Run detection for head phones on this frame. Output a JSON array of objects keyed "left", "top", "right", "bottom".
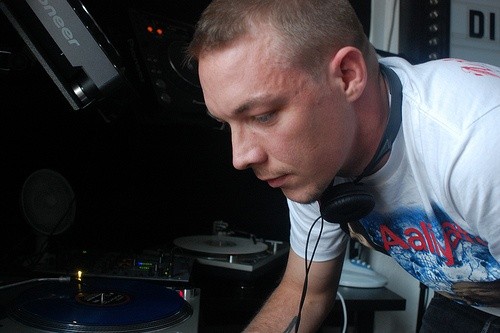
[{"left": 319, "top": 62, "right": 403, "bottom": 224}]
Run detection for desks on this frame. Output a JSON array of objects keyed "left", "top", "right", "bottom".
[{"left": 328, "top": 286, "right": 407, "bottom": 333}]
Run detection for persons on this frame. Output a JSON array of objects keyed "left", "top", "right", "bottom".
[{"left": 189, "top": 0, "right": 500, "bottom": 333}]
